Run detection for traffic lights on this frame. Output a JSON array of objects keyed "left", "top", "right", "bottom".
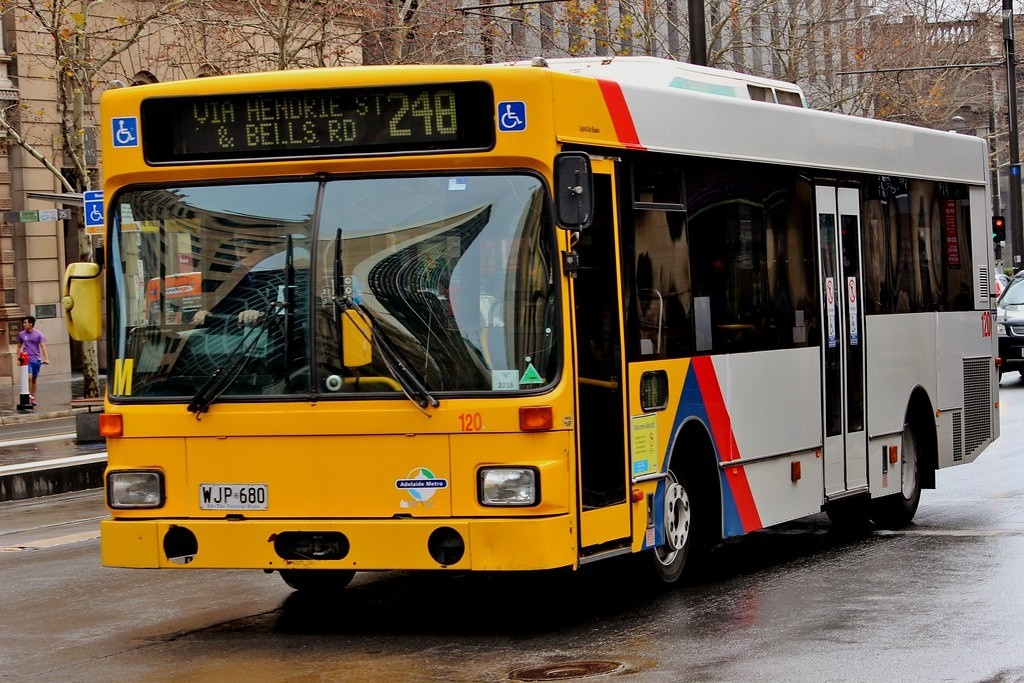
[{"left": 992, "top": 216, "right": 1006, "bottom": 242}]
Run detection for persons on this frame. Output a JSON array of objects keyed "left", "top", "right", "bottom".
[
  {"left": 16, "top": 315, "right": 49, "bottom": 405},
  {"left": 1004, "top": 268, "right": 1015, "bottom": 282},
  {"left": 192, "top": 222, "right": 288, "bottom": 329}
]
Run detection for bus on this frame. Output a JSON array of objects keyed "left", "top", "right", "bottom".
[
  {"left": 61, "top": 56, "right": 1001, "bottom": 589},
  {"left": 146, "top": 272, "right": 201, "bottom": 325}
]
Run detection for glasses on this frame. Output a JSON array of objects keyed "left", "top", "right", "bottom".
[{"left": 235, "top": 238, "right": 264, "bottom": 253}]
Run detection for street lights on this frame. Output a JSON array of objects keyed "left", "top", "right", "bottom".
[{"left": 952, "top": 116, "right": 977, "bottom": 136}]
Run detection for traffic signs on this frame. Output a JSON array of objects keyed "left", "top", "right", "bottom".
[{"left": 320, "top": 276, "right": 354, "bottom": 307}]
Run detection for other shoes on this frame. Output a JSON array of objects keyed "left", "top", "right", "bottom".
[{"left": 29, "top": 397, "right": 37, "bottom": 406}]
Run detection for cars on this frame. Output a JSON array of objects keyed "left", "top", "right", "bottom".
[{"left": 996, "top": 270, "right": 1024, "bottom": 382}]
